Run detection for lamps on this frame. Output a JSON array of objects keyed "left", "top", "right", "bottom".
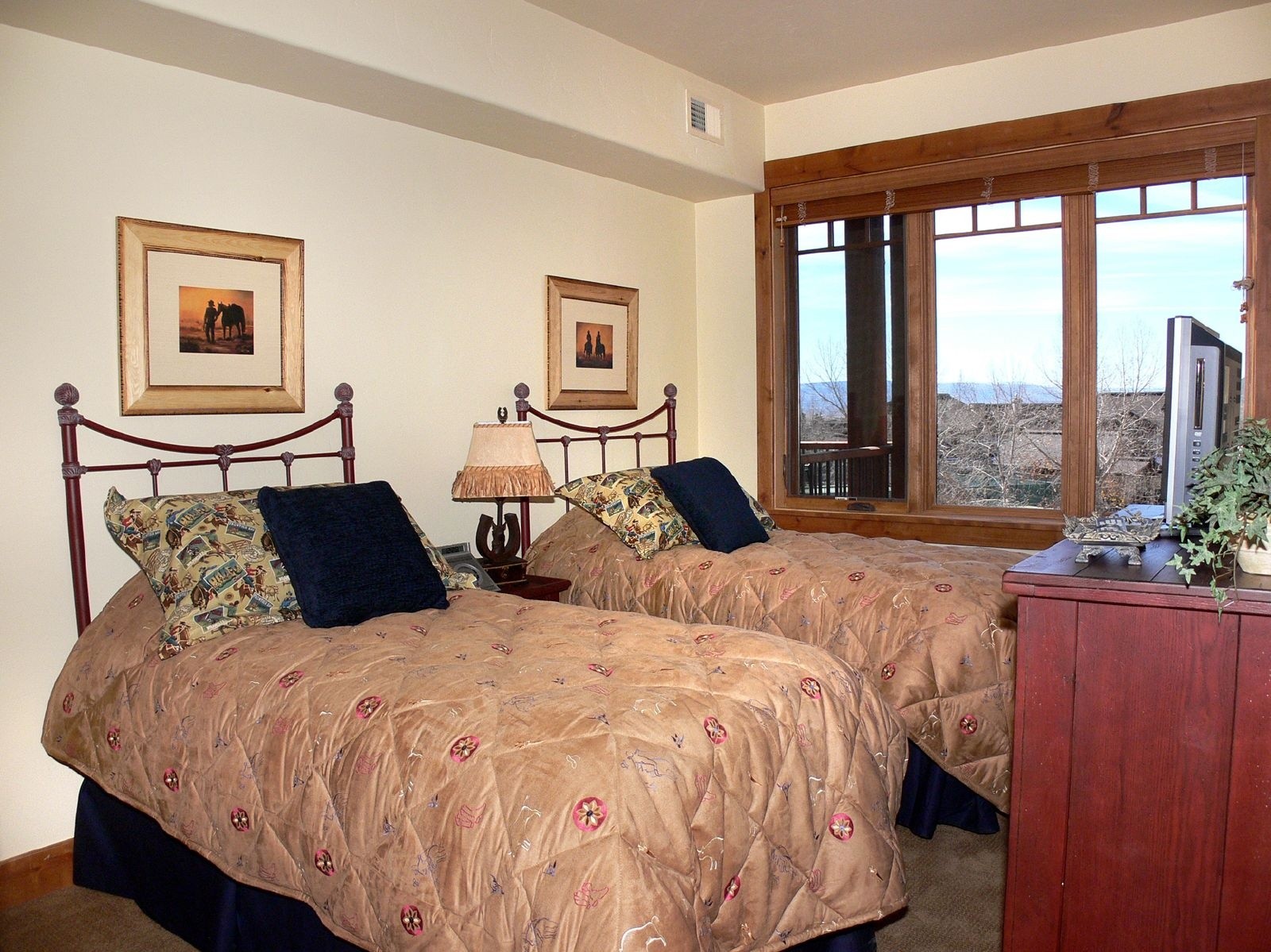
[{"left": 451, "top": 422, "right": 556, "bottom": 588}]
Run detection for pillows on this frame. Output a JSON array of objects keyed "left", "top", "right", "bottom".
[
  {"left": 556, "top": 466, "right": 779, "bottom": 556},
  {"left": 103, "top": 483, "right": 476, "bottom": 662},
  {"left": 260, "top": 482, "right": 450, "bottom": 629},
  {"left": 650, "top": 458, "right": 771, "bottom": 554}
]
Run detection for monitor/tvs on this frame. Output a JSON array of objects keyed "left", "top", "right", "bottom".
[{"left": 1147, "top": 313, "right": 1242, "bottom": 537}]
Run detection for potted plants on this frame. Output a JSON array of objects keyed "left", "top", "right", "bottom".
[{"left": 1169, "top": 419, "right": 1271, "bottom": 606}]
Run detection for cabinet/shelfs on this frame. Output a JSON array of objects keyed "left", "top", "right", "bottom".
[{"left": 1001, "top": 504, "right": 1270, "bottom": 952}]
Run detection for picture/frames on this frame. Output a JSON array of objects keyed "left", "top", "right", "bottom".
[
  {"left": 114, "top": 214, "right": 309, "bottom": 419},
  {"left": 547, "top": 276, "right": 639, "bottom": 412}
]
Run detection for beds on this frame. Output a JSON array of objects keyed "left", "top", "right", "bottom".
[
  {"left": 515, "top": 382, "right": 1044, "bottom": 841},
  {"left": 53, "top": 382, "right": 911, "bottom": 952}
]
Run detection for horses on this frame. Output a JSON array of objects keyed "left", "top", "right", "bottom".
[
  {"left": 585, "top": 335, "right": 592, "bottom": 357},
  {"left": 595, "top": 336, "right": 605, "bottom": 361},
  {"left": 216, "top": 293, "right": 246, "bottom": 339}
]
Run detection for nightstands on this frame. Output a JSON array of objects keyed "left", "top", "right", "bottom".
[{"left": 497, "top": 575, "right": 572, "bottom": 602}]
